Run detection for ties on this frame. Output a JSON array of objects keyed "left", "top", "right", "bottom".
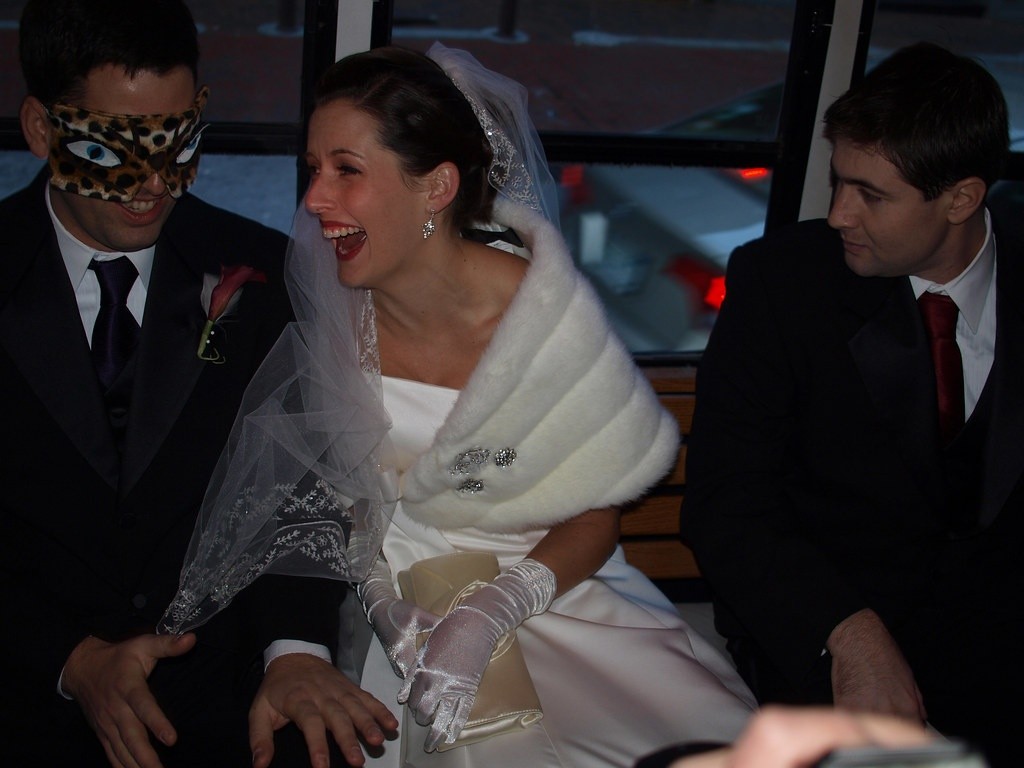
[
  {"left": 918, "top": 291, "right": 965, "bottom": 446},
  {"left": 88, "top": 256, "right": 141, "bottom": 387}
]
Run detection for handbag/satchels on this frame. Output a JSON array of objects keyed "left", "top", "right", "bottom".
[{"left": 398, "top": 551, "right": 544, "bottom": 752}]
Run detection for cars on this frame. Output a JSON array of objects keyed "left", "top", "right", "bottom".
[
  {"left": 631, "top": 50, "right": 1024, "bottom": 187},
  {"left": 536, "top": 165, "right": 771, "bottom": 361}
]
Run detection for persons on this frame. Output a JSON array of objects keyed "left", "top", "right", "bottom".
[
  {"left": 0, "top": 0, "right": 399, "bottom": 768},
  {"left": 681, "top": 43, "right": 1023, "bottom": 768},
  {"left": 168, "top": 42, "right": 759, "bottom": 768}
]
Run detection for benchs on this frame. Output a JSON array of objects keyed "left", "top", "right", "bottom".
[{"left": 613, "top": 365, "right": 704, "bottom": 578}]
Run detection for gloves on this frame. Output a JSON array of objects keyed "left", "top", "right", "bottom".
[
  {"left": 346, "top": 530, "right": 443, "bottom": 678},
  {"left": 397, "top": 558, "right": 558, "bottom": 754}
]
